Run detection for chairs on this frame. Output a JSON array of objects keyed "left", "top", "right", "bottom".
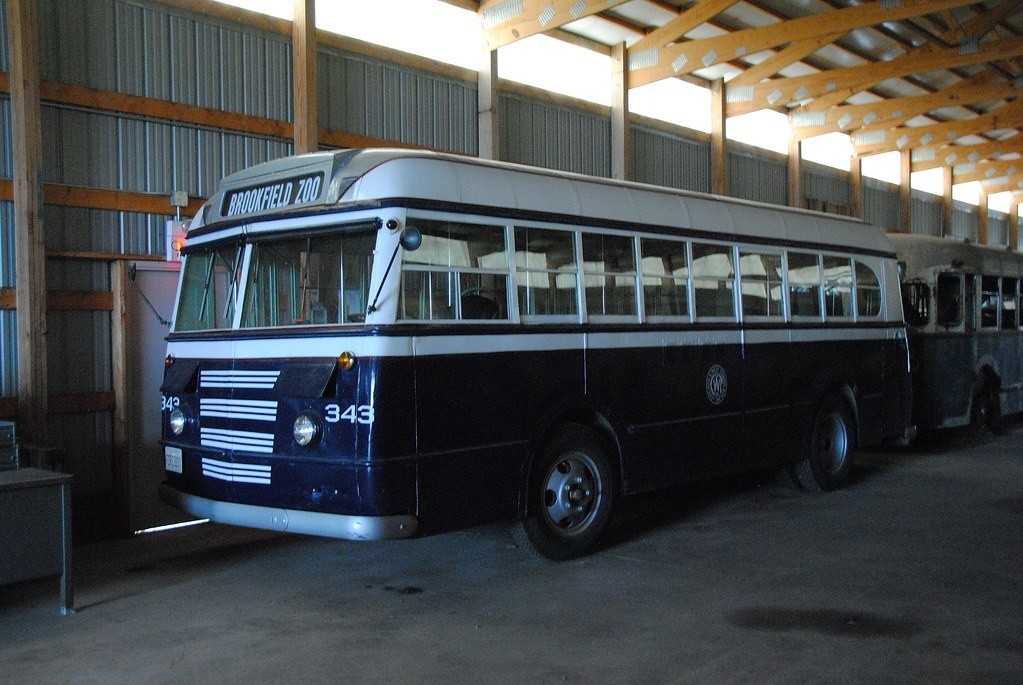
[{"left": 442, "top": 286, "right": 505, "bottom": 320}]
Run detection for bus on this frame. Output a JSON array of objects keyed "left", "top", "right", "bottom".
[
  {"left": 127, "top": 145, "right": 916, "bottom": 562},
  {"left": 884, "top": 232, "right": 1023, "bottom": 448}
]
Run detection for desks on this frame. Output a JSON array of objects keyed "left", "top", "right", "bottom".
[{"left": 0, "top": 468, "right": 76, "bottom": 616}]
